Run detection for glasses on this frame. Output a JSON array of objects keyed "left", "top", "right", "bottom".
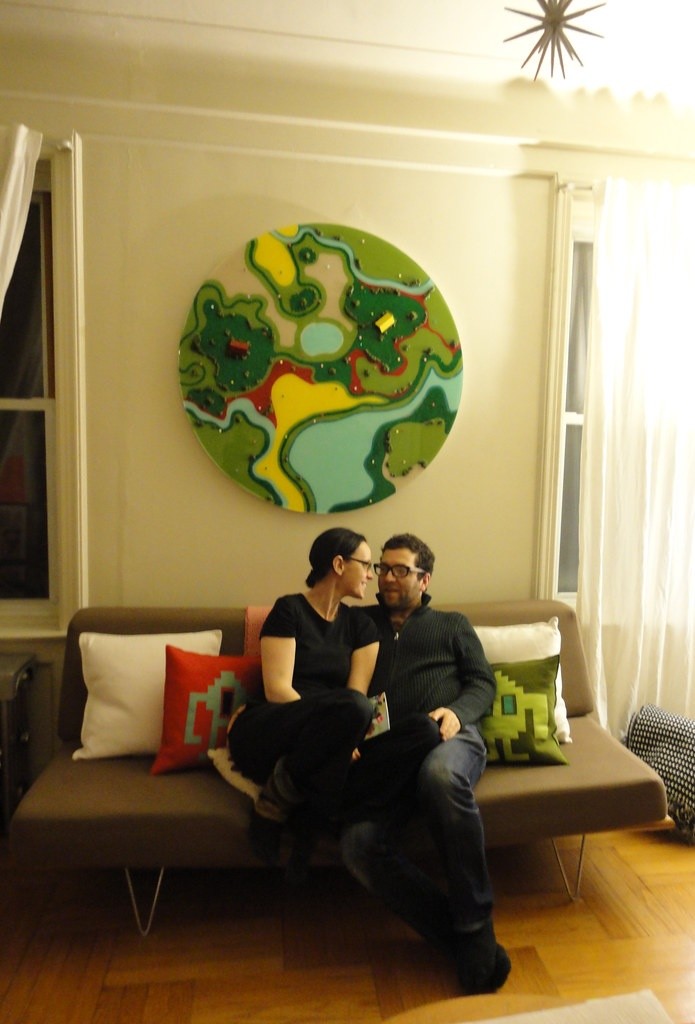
[
  {"left": 374, "top": 563, "right": 426, "bottom": 578},
  {"left": 347, "top": 557, "right": 371, "bottom": 571}
]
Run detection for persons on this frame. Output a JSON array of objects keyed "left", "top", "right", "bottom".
[
  {"left": 226, "top": 527, "right": 381, "bottom": 872},
  {"left": 226, "top": 533, "right": 511, "bottom": 993}
]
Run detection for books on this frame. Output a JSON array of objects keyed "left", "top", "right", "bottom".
[{"left": 363, "top": 692, "right": 390, "bottom": 741}]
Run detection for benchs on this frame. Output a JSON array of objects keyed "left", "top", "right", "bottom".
[{"left": 7, "top": 602, "right": 676, "bottom": 938}]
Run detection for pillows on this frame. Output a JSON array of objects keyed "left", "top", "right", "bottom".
[
  {"left": 149, "top": 645, "right": 262, "bottom": 775},
  {"left": 72, "top": 630, "right": 223, "bottom": 759},
  {"left": 474, "top": 617, "right": 573, "bottom": 763}
]
